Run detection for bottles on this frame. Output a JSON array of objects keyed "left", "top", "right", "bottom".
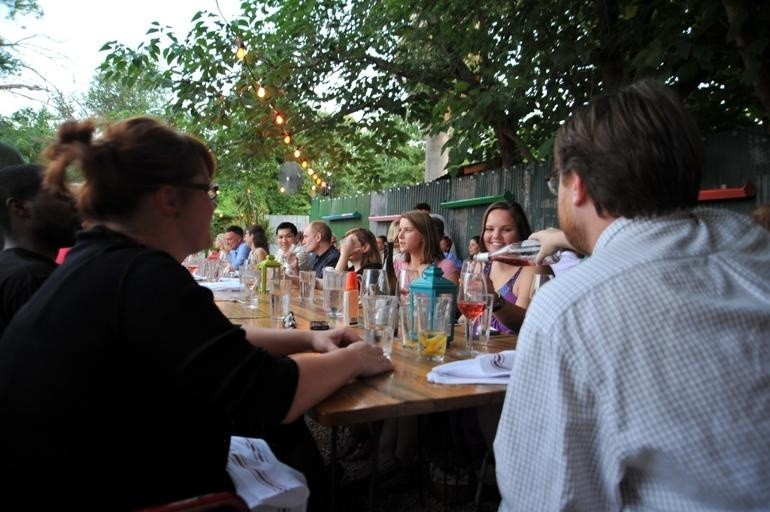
[
  {"left": 471, "top": 237, "right": 562, "bottom": 266},
  {"left": 343, "top": 272, "right": 359, "bottom": 327}
]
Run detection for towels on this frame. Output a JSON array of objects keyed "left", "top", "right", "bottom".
[
  {"left": 419, "top": 350, "right": 517, "bottom": 386},
  {"left": 225, "top": 433, "right": 312, "bottom": 512}
]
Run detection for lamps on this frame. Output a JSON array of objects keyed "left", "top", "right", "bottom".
[{"left": 409, "top": 262, "right": 458, "bottom": 348}]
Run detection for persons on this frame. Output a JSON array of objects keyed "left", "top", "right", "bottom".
[
  {"left": 0, "top": 114, "right": 396, "bottom": 511},
  {"left": 215, "top": 204, "right": 462, "bottom": 322},
  {"left": 490, "top": 79, "right": 770, "bottom": 512},
  {"left": 0, "top": 141, "right": 89, "bottom": 328},
  {"left": 459, "top": 200, "right": 549, "bottom": 339}
]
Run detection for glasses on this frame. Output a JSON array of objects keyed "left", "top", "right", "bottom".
[
  {"left": 544, "top": 170, "right": 559, "bottom": 197},
  {"left": 169, "top": 180, "right": 219, "bottom": 200}
]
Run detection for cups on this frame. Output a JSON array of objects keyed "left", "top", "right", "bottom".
[
  {"left": 469, "top": 291, "right": 494, "bottom": 352},
  {"left": 186, "top": 250, "right": 341, "bottom": 324},
  {"left": 363, "top": 292, "right": 453, "bottom": 365}
]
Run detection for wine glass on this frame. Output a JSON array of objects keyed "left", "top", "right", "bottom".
[
  {"left": 457, "top": 273, "right": 485, "bottom": 358},
  {"left": 395, "top": 268, "right": 421, "bottom": 302},
  {"left": 360, "top": 268, "right": 390, "bottom": 298}
]
